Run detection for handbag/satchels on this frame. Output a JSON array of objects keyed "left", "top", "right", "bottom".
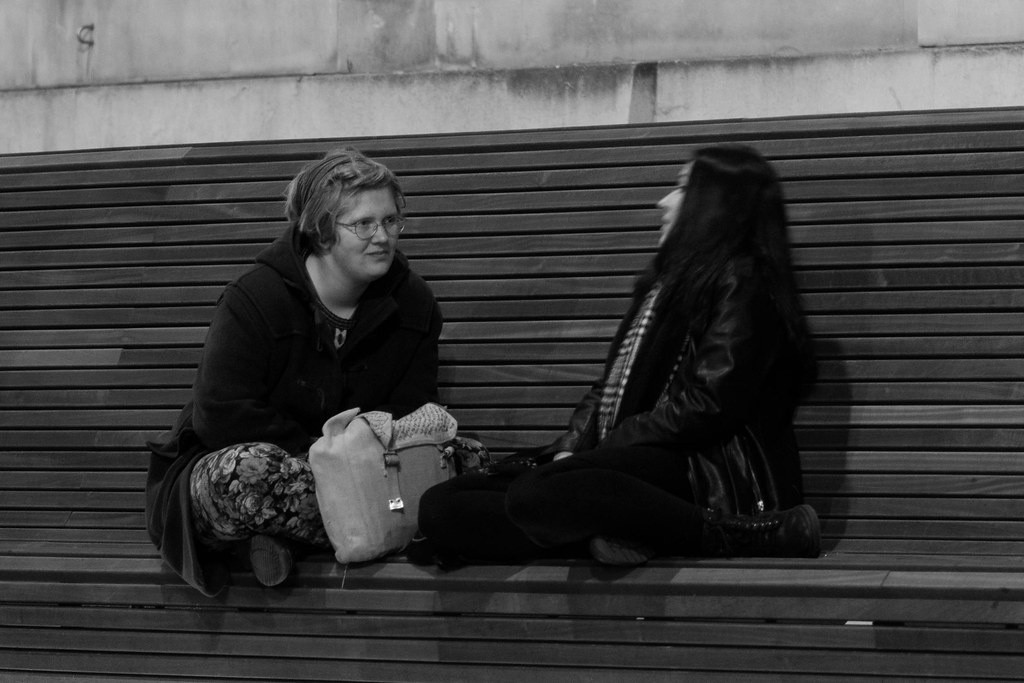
[{"left": 308, "top": 401, "right": 459, "bottom": 565}]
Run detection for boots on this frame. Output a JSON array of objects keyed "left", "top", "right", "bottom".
[
  {"left": 697, "top": 497, "right": 821, "bottom": 559},
  {"left": 587, "top": 536, "right": 647, "bottom": 566}
]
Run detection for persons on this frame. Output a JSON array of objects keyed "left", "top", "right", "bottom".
[
  {"left": 146, "top": 145, "right": 443, "bottom": 597},
  {"left": 543, "top": 146, "right": 822, "bottom": 563}
]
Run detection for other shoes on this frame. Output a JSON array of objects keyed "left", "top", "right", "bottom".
[{"left": 250, "top": 534, "right": 293, "bottom": 587}]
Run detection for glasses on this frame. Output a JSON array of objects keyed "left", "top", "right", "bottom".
[{"left": 335, "top": 214, "right": 408, "bottom": 240}]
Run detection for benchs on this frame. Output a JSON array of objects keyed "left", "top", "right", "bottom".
[{"left": 0, "top": 106, "right": 1024, "bottom": 683}]
